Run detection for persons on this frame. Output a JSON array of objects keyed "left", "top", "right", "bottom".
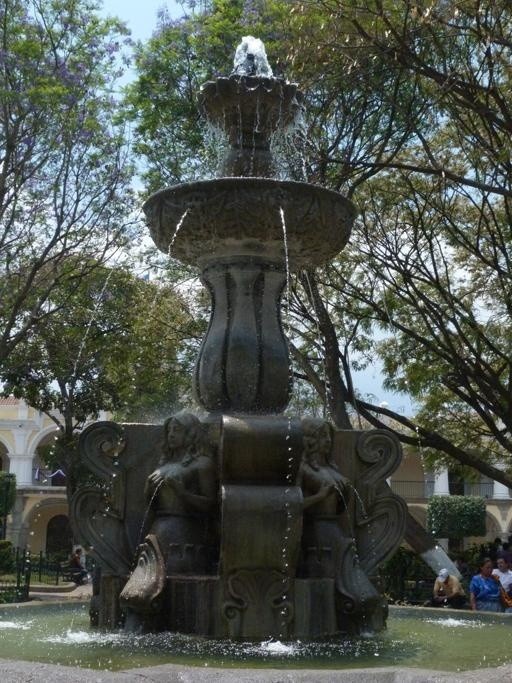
[
  {"left": 423, "top": 567, "right": 466, "bottom": 609},
  {"left": 295, "top": 417, "right": 379, "bottom": 632},
  {"left": 69, "top": 547, "right": 84, "bottom": 569},
  {"left": 118, "top": 411, "right": 217, "bottom": 634},
  {"left": 469, "top": 557, "right": 511, "bottom": 611},
  {"left": 491, "top": 556, "right": 512, "bottom": 612}
]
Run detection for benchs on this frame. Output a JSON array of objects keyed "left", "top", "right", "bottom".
[{"left": 60, "top": 555, "right": 93, "bottom": 586}]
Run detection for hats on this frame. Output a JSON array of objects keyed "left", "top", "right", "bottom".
[{"left": 438, "top": 568, "right": 450, "bottom": 583}]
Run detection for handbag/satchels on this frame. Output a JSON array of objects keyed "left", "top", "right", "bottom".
[{"left": 498, "top": 585, "right": 512, "bottom": 609}]
